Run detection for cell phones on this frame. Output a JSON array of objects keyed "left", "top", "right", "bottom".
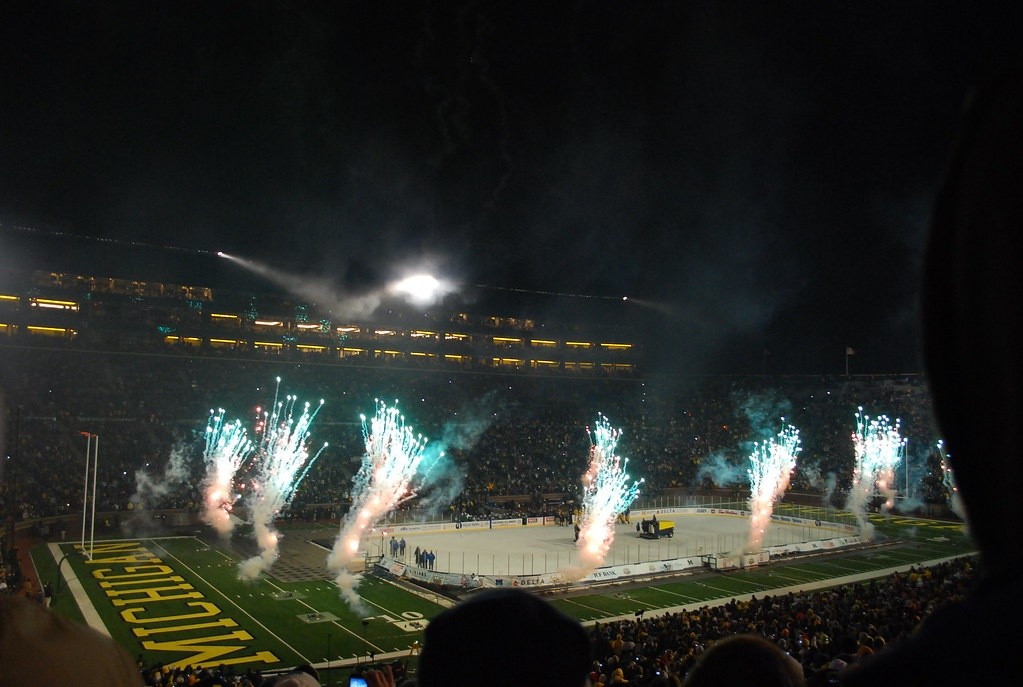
[{"left": 347, "top": 674, "right": 368, "bottom": 687}]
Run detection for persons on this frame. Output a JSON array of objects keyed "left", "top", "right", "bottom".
[{"left": 0, "top": 90, "right": 1022, "bottom": 687}]
